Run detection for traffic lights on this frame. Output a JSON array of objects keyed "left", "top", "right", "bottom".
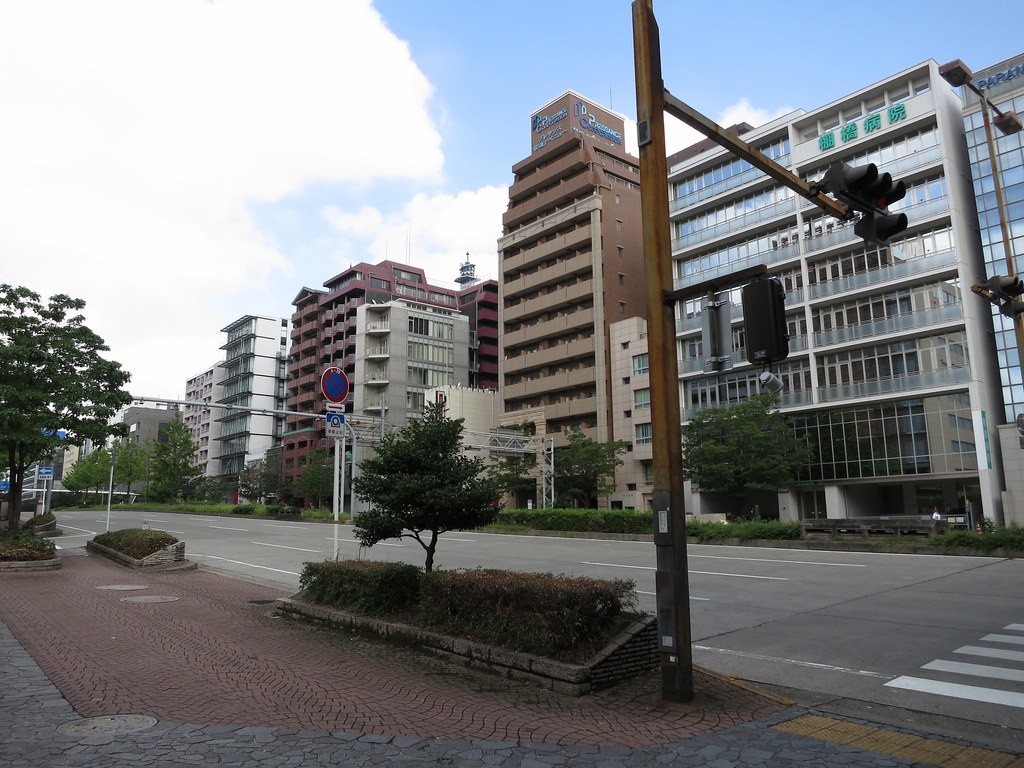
[
  {"left": 994, "top": 275, "right": 1024, "bottom": 301},
  {"left": 837, "top": 159, "right": 907, "bottom": 212},
  {"left": 869, "top": 211, "right": 908, "bottom": 247},
  {"left": 1011, "top": 301, "right": 1024, "bottom": 318}
]
